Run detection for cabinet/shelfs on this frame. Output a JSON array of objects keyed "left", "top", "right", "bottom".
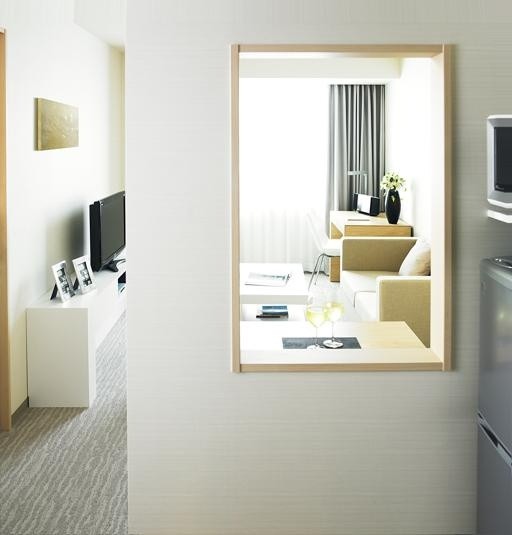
[{"left": 27, "top": 250, "right": 126, "bottom": 409}]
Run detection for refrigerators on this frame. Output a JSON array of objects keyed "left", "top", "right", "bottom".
[{"left": 477, "top": 255, "right": 512, "bottom": 535}]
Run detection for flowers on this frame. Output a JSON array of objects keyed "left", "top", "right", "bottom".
[{"left": 379, "top": 171, "right": 407, "bottom": 196}]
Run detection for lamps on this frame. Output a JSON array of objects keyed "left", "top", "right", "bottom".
[{"left": 347, "top": 171, "right": 369, "bottom": 195}]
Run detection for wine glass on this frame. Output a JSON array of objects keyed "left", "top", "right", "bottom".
[{"left": 304, "top": 297, "right": 345, "bottom": 350}]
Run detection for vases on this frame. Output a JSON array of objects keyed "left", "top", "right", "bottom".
[{"left": 385, "top": 189, "right": 401, "bottom": 225}]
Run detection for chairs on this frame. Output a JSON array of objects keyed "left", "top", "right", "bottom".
[{"left": 307, "top": 214, "right": 340, "bottom": 289}]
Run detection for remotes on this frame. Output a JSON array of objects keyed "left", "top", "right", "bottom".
[{"left": 256, "top": 315, "right": 280, "bottom": 318}]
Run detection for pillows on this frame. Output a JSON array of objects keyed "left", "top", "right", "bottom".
[{"left": 395, "top": 236, "right": 432, "bottom": 277}]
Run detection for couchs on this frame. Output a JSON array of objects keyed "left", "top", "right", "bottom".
[{"left": 335, "top": 236, "right": 431, "bottom": 346}]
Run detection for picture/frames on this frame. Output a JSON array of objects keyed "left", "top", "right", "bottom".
[{"left": 49, "top": 254, "right": 98, "bottom": 302}]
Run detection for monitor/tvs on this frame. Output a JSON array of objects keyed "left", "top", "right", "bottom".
[{"left": 90, "top": 191, "right": 126, "bottom": 274}]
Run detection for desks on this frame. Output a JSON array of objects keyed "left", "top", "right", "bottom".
[
  {"left": 240, "top": 320, "right": 427, "bottom": 353},
  {"left": 328, "top": 211, "right": 412, "bottom": 283}
]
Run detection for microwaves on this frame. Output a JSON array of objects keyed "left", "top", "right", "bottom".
[{"left": 484, "top": 114, "right": 512, "bottom": 225}]
